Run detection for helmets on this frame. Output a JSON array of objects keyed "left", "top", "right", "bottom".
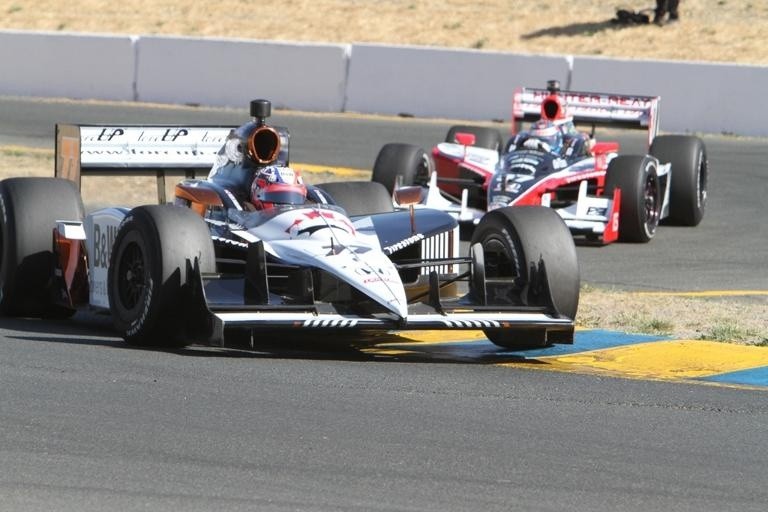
[{"left": 530, "top": 120, "right": 557, "bottom": 144}]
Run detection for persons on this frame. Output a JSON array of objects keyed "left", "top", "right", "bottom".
[
  {"left": 653, "top": 1, "right": 680, "bottom": 27},
  {"left": 522, "top": 119, "right": 565, "bottom": 161}
]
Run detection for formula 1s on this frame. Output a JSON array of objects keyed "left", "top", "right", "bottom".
[
  {"left": 1, "top": 99, "right": 580, "bottom": 351},
  {"left": 370, "top": 79, "right": 710, "bottom": 245}
]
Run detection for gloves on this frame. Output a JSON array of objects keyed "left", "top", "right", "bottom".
[{"left": 524, "top": 138, "right": 542, "bottom": 149}]
